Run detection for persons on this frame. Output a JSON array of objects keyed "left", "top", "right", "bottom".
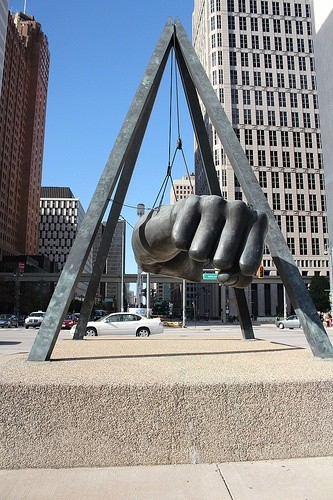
[{"left": 318, "top": 310, "right": 332, "bottom": 327}]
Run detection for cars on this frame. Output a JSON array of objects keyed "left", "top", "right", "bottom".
[
  {"left": 276, "top": 314, "right": 303, "bottom": 329},
  {"left": 70, "top": 312, "right": 165, "bottom": 337},
  {"left": 0, "top": 314, "right": 19, "bottom": 328},
  {"left": 61, "top": 314, "right": 77, "bottom": 329}
]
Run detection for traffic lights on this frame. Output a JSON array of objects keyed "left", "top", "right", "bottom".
[{"left": 258, "top": 266, "right": 264, "bottom": 278}]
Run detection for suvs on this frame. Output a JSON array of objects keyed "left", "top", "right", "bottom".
[{"left": 24, "top": 311, "right": 46, "bottom": 329}]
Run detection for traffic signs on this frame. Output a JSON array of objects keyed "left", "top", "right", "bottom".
[{"left": 203, "top": 273, "right": 217, "bottom": 280}]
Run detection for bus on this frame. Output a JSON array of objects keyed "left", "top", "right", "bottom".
[{"left": 94, "top": 310, "right": 104, "bottom": 319}]
